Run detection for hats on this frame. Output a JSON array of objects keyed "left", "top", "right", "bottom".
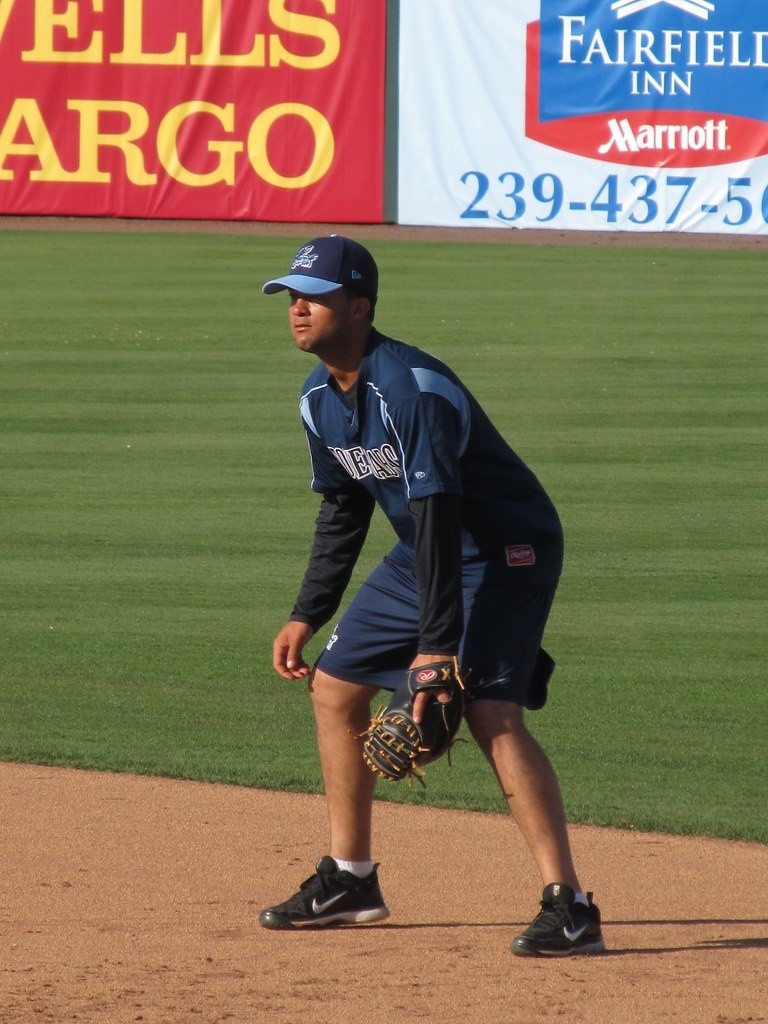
[{"left": 262, "top": 234, "right": 379, "bottom": 296}]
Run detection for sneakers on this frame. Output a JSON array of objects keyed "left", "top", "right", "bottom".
[
  {"left": 259, "top": 856, "right": 390, "bottom": 931},
  {"left": 512, "top": 882, "right": 606, "bottom": 957}
]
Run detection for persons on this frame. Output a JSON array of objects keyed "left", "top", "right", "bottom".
[{"left": 258, "top": 233, "right": 608, "bottom": 956}]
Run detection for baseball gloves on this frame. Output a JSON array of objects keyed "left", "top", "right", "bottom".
[{"left": 361, "top": 658, "right": 464, "bottom": 782}]
